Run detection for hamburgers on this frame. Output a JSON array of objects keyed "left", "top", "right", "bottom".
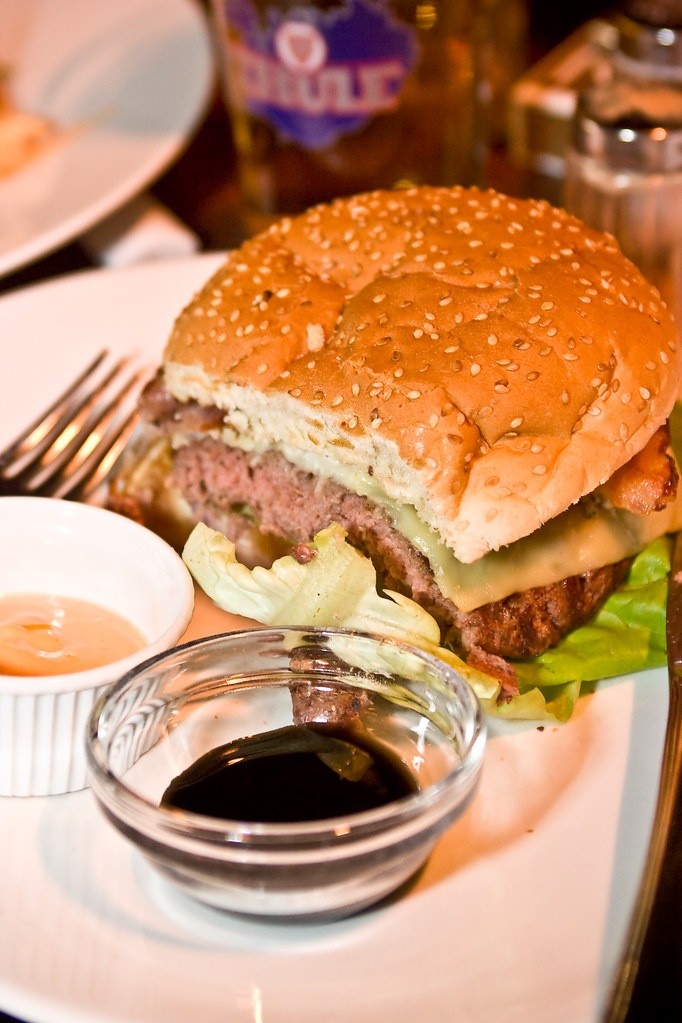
[{"left": 137, "top": 186, "right": 682, "bottom": 730}]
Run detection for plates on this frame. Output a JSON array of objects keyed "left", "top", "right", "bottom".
[
  {"left": 0, "top": 0, "right": 217, "bottom": 277},
  {"left": 0, "top": 251, "right": 669, "bottom": 1023}
]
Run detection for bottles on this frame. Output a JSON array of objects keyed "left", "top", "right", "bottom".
[
  {"left": 205, "top": 0, "right": 531, "bottom": 238},
  {"left": 510, "top": 0, "right": 682, "bottom": 322}
]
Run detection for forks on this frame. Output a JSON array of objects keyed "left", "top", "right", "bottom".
[{"left": 1, "top": 348, "right": 165, "bottom": 504}]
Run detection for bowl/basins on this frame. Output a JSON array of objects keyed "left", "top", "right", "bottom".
[
  {"left": 1, "top": 494, "right": 196, "bottom": 798},
  {"left": 85, "top": 626, "right": 488, "bottom": 923}
]
